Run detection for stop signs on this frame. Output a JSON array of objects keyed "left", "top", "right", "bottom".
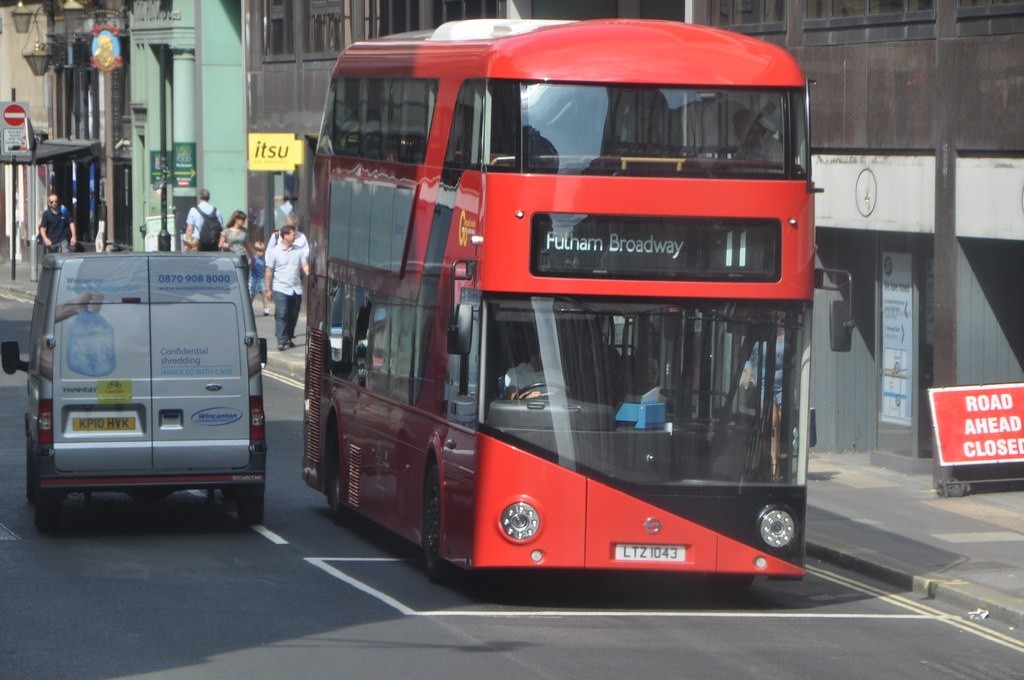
[{"left": 1, "top": 101, "right": 32, "bottom": 162}]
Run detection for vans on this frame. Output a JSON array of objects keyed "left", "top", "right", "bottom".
[{"left": 0, "top": 251, "right": 271, "bottom": 536}]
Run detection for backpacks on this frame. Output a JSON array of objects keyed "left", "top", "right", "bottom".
[{"left": 194, "top": 205, "right": 222, "bottom": 251}]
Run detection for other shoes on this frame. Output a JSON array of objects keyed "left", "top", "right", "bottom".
[
  {"left": 285, "top": 340, "right": 295, "bottom": 347},
  {"left": 278, "top": 344, "right": 285, "bottom": 351},
  {"left": 263, "top": 308, "right": 269, "bottom": 316}
]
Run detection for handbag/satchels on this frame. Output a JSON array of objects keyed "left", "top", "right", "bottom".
[{"left": 37, "top": 234, "right": 43, "bottom": 245}]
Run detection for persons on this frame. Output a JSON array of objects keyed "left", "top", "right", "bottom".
[
  {"left": 218, "top": 209, "right": 254, "bottom": 284},
  {"left": 39, "top": 193, "right": 77, "bottom": 253},
  {"left": 245, "top": 242, "right": 271, "bottom": 316},
  {"left": 55, "top": 291, "right": 104, "bottom": 323},
  {"left": 742, "top": 324, "right": 787, "bottom": 477},
  {"left": 184, "top": 192, "right": 224, "bottom": 253},
  {"left": 638, "top": 357, "right": 660, "bottom": 387},
  {"left": 264, "top": 216, "right": 310, "bottom": 349},
  {"left": 501, "top": 347, "right": 565, "bottom": 398}
]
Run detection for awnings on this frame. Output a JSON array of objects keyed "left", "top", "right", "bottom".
[{"left": 0, "top": 137, "right": 99, "bottom": 279}]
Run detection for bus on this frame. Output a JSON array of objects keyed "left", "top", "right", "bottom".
[{"left": 303, "top": 19, "right": 856, "bottom": 594}]
